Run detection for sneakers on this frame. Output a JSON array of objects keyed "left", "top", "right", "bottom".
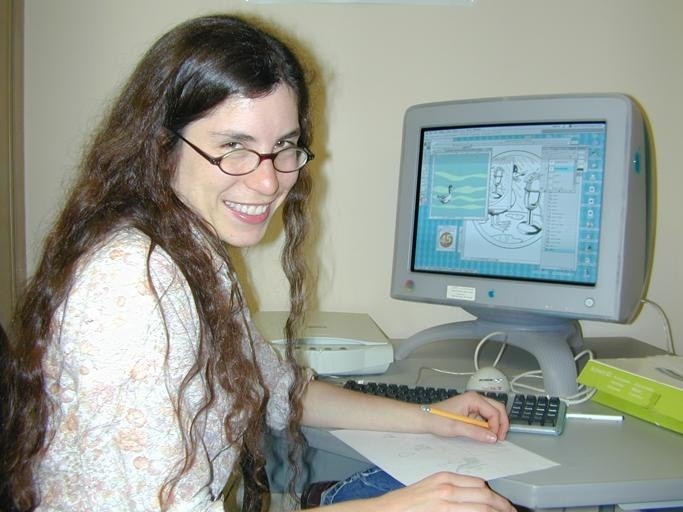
[{"left": 301, "top": 481, "right": 341, "bottom": 509}]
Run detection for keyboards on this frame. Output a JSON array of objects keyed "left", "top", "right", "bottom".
[{"left": 340, "top": 379, "right": 567, "bottom": 434}]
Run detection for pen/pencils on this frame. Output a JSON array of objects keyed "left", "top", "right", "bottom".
[
  {"left": 417, "top": 404, "right": 489, "bottom": 427},
  {"left": 566, "top": 413, "right": 625, "bottom": 421}
]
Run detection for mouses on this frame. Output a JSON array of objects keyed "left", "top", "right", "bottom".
[{"left": 466, "top": 363, "right": 512, "bottom": 395}]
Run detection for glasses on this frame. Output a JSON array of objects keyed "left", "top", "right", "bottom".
[{"left": 162, "top": 123, "right": 314, "bottom": 177}]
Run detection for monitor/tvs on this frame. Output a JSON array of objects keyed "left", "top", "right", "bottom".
[{"left": 390, "top": 91, "right": 656, "bottom": 399}]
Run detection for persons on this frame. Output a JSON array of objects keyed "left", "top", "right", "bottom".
[{"left": 10, "top": 12, "right": 516, "bottom": 511}]
